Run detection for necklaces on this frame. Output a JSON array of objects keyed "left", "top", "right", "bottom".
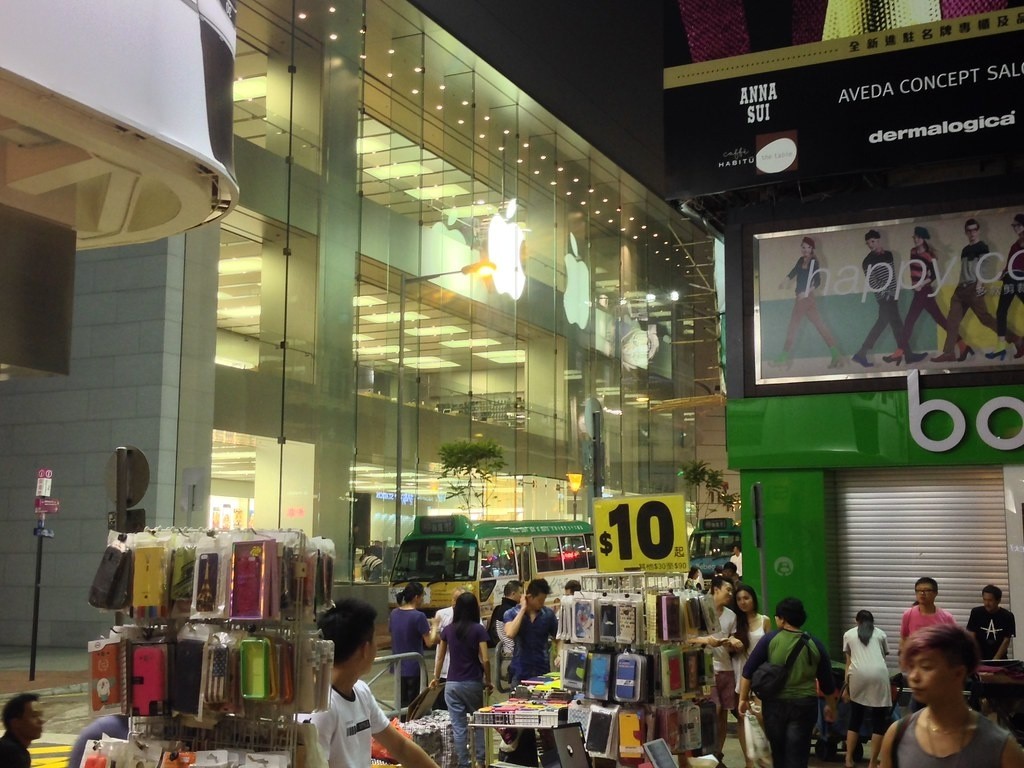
[{"left": 926, "top": 710, "right": 971, "bottom": 735}]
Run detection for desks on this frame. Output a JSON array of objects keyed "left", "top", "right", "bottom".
[{"left": 983, "top": 674, "right": 1024, "bottom": 726}]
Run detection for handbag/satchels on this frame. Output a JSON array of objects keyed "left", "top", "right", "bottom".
[
  {"left": 751, "top": 664, "right": 788, "bottom": 704},
  {"left": 743, "top": 709, "right": 772, "bottom": 761}
]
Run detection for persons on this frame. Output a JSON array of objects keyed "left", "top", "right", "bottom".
[
  {"left": 557, "top": 580, "right": 582, "bottom": 621},
  {"left": 294, "top": 599, "right": 441, "bottom": 768},
  {"left": 0, "top": 692, "right": 46, "bottom": 768},
  {"left": 388, "top": 582, "right": 441, "bottom": 721},
  {"left": 493, "top": 578, "right": 559, "bottom": 700},
  {"left": 429, "top": 589, "right": 495, "bottom": 768},
  {"left": 684, "top": 562, "right": 1024, "bottom": 768},
  {"left": 70, "top": 714, "right": 129, "bottom": 768},
  {"left": 768, "top": 213, "right": 1024, "bottom": 370},
  {"left": 729, "top": 544, "right": 742, "bottom": 581}
]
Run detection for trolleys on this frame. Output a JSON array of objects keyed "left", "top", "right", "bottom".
[{"left": 814, "top": 669, "right": 904, "bottom": 758}]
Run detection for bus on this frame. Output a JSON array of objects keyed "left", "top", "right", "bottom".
[
  {"left": 386, "top": 515, "right": 595, "bottom": 614},
  {"left": 687, "top": 517, "right": 742, "bottom": 570}
]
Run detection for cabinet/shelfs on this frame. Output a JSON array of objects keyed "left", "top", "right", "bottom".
[{"left": 452, "top": 402, "right": 525, "bottom": 429}]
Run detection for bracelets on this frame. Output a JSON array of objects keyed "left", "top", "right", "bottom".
[{"left": 435, "top": 677, "right": 440, "bottom": 681}]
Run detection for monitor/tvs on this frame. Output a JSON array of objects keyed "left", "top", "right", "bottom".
[{"left": 552, "top": 727, "right": 589, "bottom": 768}]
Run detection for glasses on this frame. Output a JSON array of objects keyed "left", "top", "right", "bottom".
[{"left": 916, "top": 590, "right": 934, "bottom": 594}]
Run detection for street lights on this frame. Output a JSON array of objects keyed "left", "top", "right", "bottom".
[{"left": 567, "top": 472, "right": 583, "bottom": 521}]
